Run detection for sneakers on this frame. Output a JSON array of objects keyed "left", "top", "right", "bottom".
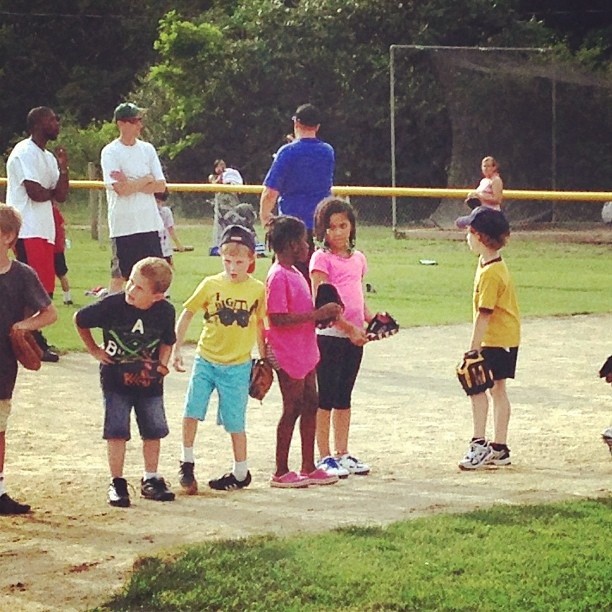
[
  {"left": 457, "top": 440, "right": 494, "bottom": 470},
  {"left": 107, "top": 477, "right": 135, "bottom": 507},
  {"left": 336, "top": 454, "right": 369, "bottom": 475},
  {"left": 210, "top": 469, "right": 251, "bottom": 490},
  {"left": 42, "top": 346, "right": 59, "bottom": 361},
  {"left": 316, "top": 455, "right": 350, "bottom": 478},
  {"left": 178, "top": 460, "right": 197, "bottom": 495},
  {"left": 300, "top": 468, "right": 338, "bottom": 485},
  {"left": 270, "top": 471, "right": 310, "bottom": 488},
  {"left": 141, "top": 476, "right": 175, "bottom": 501},
  {"left": 484, "top": 443, "right": 511, "bottom": 465},
  {"left": 0, "top": 493, "right": 30, "bottom": 514}
]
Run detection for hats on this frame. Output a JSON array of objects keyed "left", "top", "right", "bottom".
[
  {"left": 221, "top": 224, "right": 256, "bottom": 274},
  {"left": 456, "top": 205, "right": 510, "bottom": 240},
  {"left": 115, "top": 104, "right": 149, "bottom": 121},
  {"left": 291, "top": 103, "right": 320, "bottom": 126}
]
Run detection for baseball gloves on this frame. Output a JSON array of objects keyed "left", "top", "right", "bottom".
[
  {"left": 9, "top": 327, "right": 44, "bottom": 371},
  {"left": 315, "top": 284, "right": 345, "bottom": 330},
  {"left": 249, "top": 358, "right": 273, "bottom": 400},
  {"left": 366, "top": 311, "right": 399, "bottom": 341},
  {"left": 113, "top": 359, "right": 169, "bottom": 392},
  {"left": 456, "top": 350, "right": 494, "bottom": 396}
]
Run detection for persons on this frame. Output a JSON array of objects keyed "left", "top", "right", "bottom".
[
  {"left": 307, "top": 197, "right": 401, "bottom": 481},
  {"left": 258, "top": 101, "right": 336, "bottom": 297},
  {"left": 170, "top": 223, "right": 271, "bottom": 496},
  {"left": 153, "top": 184, "right": 186, "bottom": 298},
  {"left": 456, "top": 205, "right": 522, "bottom": 472},
  {"left": 5, "top": 105, "right": 71, "bottom": 367},
  {"left": 261, "top": 214, "right": 343, "bottom": 489},
  {"left": 98, "top": 102, "right": 167, "bottom": 297},
  {"left": 597, "top": 355, "right": 612, "bottom": 456},
  {"left": 51, "top": 196, "right": 73, "bottom": 306},
  {"left": 206, "top": 155, "right": 242, "bottom": 249},
  {"left": 0, "top": 205, "right": 59, "bottom": 517},
  {"left": 72, "top": 256, "right": 177, "bottom": 509},
  {"left": 464, "top": 155, "right": 504, "bottom": 212}
]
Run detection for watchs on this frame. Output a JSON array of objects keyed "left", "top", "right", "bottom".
[{"left": 58, "top": 166, "right": 71, "bottom": 175}]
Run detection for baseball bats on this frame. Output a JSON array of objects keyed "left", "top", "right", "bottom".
[{"left": 173, "top": 246, "right": 194, "bottom": 251}]
